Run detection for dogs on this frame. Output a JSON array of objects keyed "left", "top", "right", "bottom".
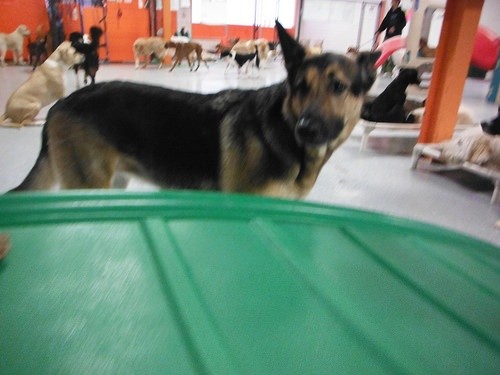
[
  {"left": 442, "top": 126, "right": 499, "bottom": 227},
  {"left": 9, "top": 20, "right": 383, "bottom": 201},
  {"left": 1, "top": 19, "right": 422, "bottom": 129}
]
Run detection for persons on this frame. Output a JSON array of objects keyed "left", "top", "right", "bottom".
[
  {"left": 376, "top": 0, "right": 407, "bottom": 74},
  {"left": 44, "top": 0, "right": 67, "bottom": 54},
  {"left": 72, "top": 25, "right": 103, "bottom": 85},
  {"left": 68, "top": 30, "right": 85, "bottom": 74}
]
